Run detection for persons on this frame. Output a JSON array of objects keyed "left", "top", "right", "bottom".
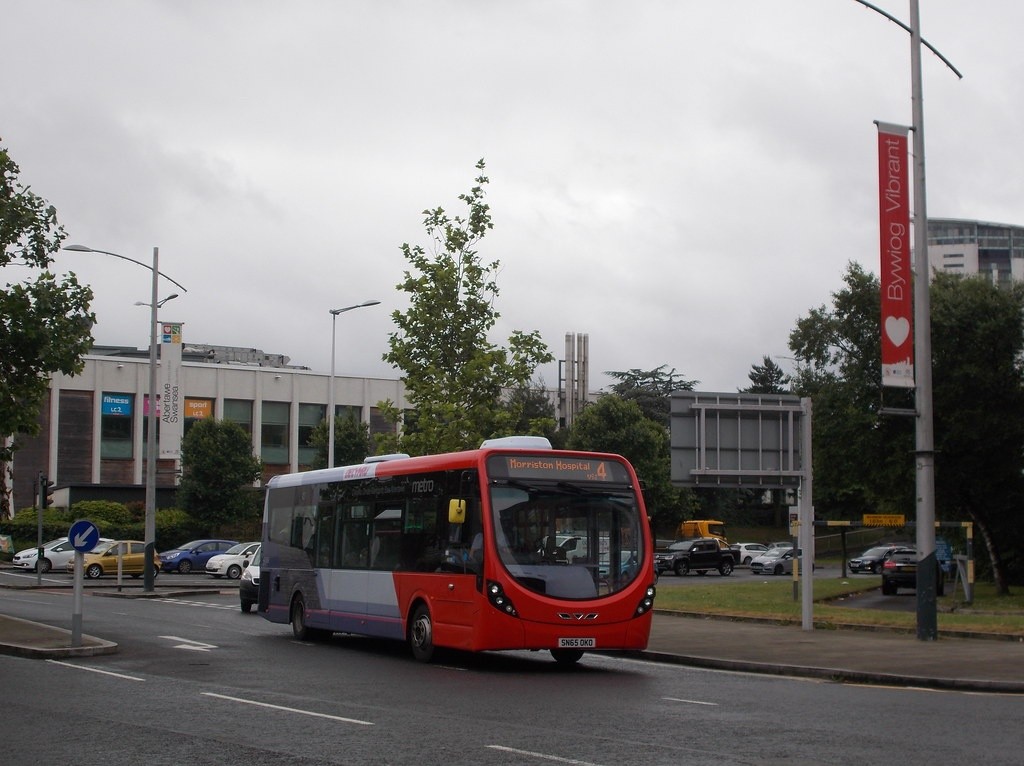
[{"left": 471, "top": 528, "right": 514, "bottom": 573}]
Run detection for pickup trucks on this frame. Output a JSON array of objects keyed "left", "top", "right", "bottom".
[{"left": 652, "top": 537, "right": 740, "bottom": 579}]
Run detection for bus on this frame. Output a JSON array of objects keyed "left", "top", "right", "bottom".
[{"left": 256, "top": 437, "right": 659, "bottom": 668}]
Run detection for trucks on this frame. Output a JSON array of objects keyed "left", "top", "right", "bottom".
[
  {"left": 534, "top": 534, "right": 610, "bottom": 562},
  {"left": 652, "top": 519, "right": 729, "bottom": 553}
]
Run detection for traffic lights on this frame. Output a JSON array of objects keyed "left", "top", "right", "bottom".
[{"left": 41, "top": 477, "right": 55, "bottom": 509}]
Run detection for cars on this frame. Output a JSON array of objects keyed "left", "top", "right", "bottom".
[
  {"left": 768, "top": 541, "right": 794, "bottom": 552},
  {"left": 735, "top": 543, "right": 772, "bottom": 566},
  {"left": 848, "top": 544, "right": 911, "bottom": 573},
  {"left": 239, "top": 544, "right": 261, "bottom": 613},
  {"left": 67, "top": 540, "right": 162, "bottom": 579},
  {"left": 203, "top": 541, "right": 261, "bottom": 579},
  {"left": 881, "top": 546, "right": 946, "bottom": 596},
  {"left": 12, "top": 535, "right": 116, "bottom": 573},
  {"left": 597, "top": 550, "right": 659, "bottom": 586},
  {"left": 158, "top": 540, "right": 240, "bottom": 575},
  {"left": 750, "top": 547, "right": 815, "bottom": 575}
]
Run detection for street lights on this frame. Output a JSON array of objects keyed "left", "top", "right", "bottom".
[
  {"left": 60, "top": 242, "right": 189, "bottom": 593},
  {"left": 328, "top": 300, "right": 382, "bottom": 468}
]
